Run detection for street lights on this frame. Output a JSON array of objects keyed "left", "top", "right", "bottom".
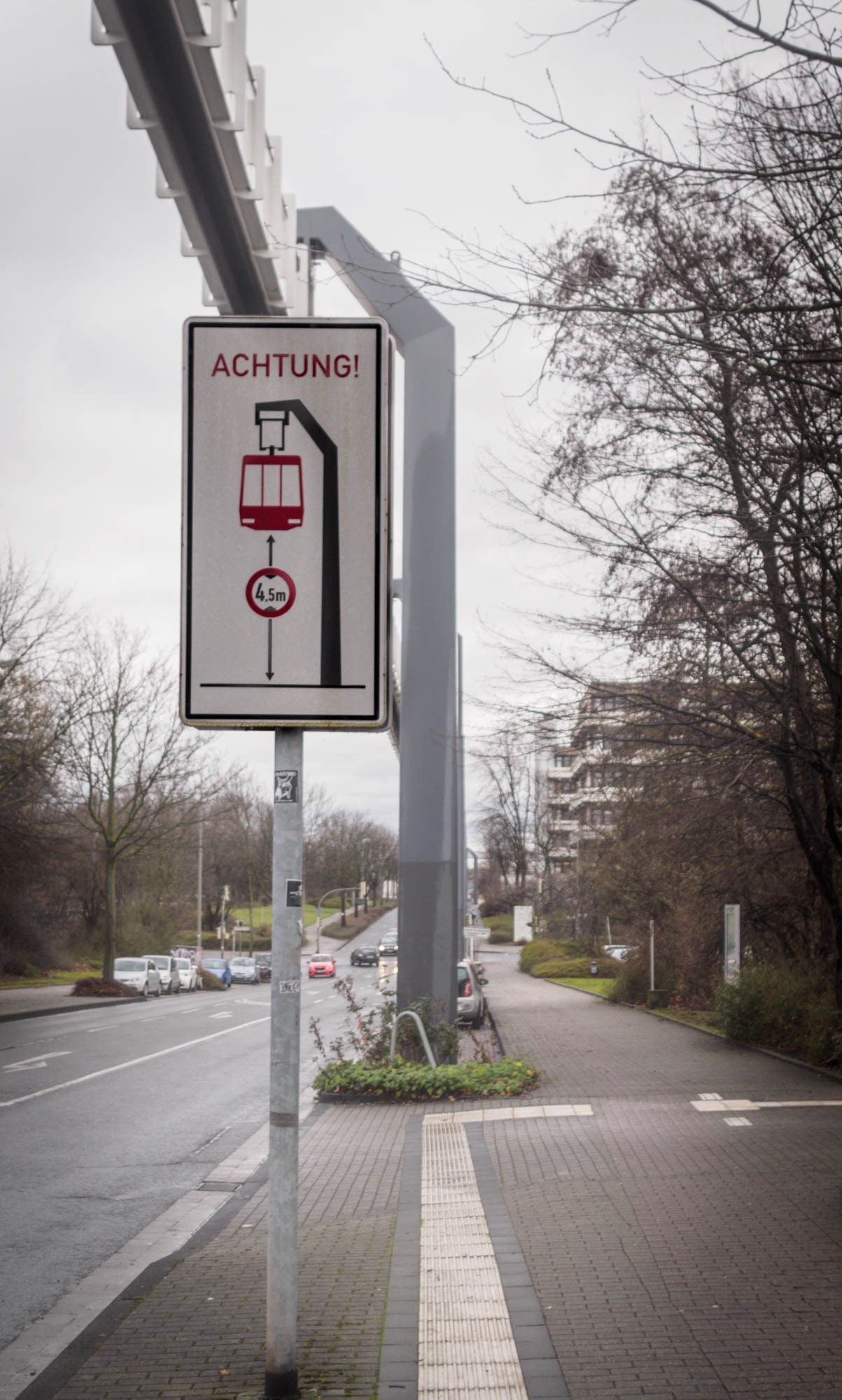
[
  {"left": 315, "top": 887, "right": 362, "bottom": 953},
  {"left": 360, "top": 837, "right": 374, "bottom": 922}
]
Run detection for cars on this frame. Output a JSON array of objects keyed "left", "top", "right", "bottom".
[
  {"left": 306, "top": 953, "right": 336, "bottom": 979},
  {"left": 252, "top": 953, "right": 272, "bottom": 982},
  {"left": 142, "top": 954, "right": 181, "bottom": 996},
  {"left": 457, "top": 959, "right": 490, "bottom": 1030},
  {"left": 378, "top": 932, "right": 398, "bottom": 956},
  {"left": 228, "top": 956, "right": 263, "bottom": 984},
  {"left": 113, "top": 957, "right": 163, "bottom": 999},
  {"left": 351, "top": 946, "right": 380, "bottom": 967},
  {"left": 174, "top": 957, "right": 198, "bottom": 994},
  {"left": 200, "top": 958, "right": 233, "bottom": 988}
]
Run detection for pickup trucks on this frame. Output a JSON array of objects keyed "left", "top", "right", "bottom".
[{"left": 218, "top": 919, "right": 251, "bottom": 934}]
